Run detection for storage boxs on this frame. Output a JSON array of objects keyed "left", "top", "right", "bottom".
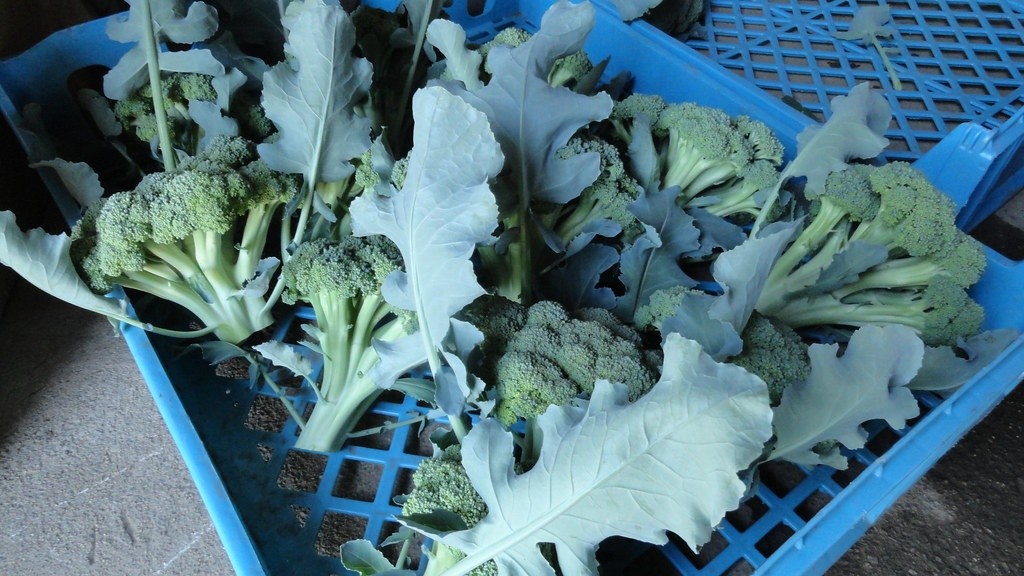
[
  {"left": 1, "top": 0, "right": 1023, "bottom": 576},
  {"left": 590, "top": 1, "right": 1024, "bottom": 236}
]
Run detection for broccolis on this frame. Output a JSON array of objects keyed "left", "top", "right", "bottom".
[{"left": 0, "top": 0, "right": 1015, "bottom": 575}]
